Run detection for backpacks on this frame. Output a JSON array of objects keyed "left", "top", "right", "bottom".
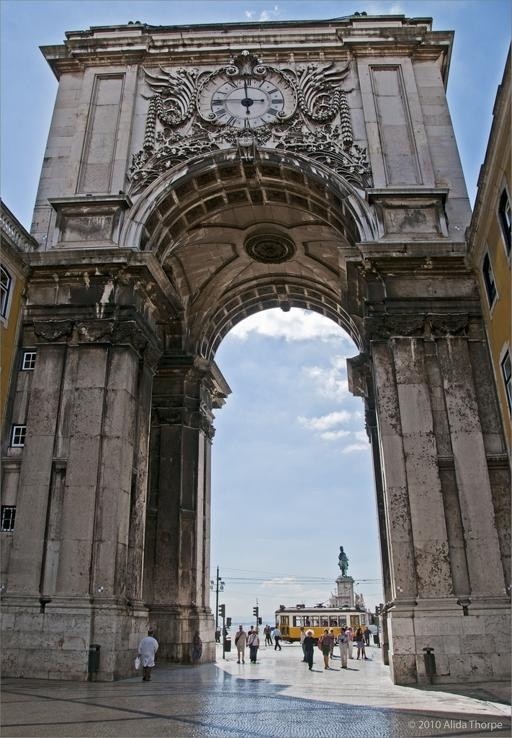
[{"left": 318, "top": 634, "right": 324, "bottom": 649}]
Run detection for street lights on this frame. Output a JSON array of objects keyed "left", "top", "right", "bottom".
[{"left": 210, "top": 567, "right": 224, "bottom": 630}]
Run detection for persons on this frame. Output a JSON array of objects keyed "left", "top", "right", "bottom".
[
  {"left": 217, "top": 625, "right": 282, "bottom": 664},
  {"left": 138, "top": 629, "right": 159, "bottom": 681},
  {"left": 300, "top": 617, "right": 372, "bottom": 670},
  {"left": 338, "top": 546, "right": 349, "bottom": 578},
  {"left": 193, "top": 629, "right": 202, "bottom": 667}
]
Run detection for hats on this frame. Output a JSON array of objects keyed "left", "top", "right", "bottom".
[{"left": 306, "top": 629, "right": 314, "bottom": 637}]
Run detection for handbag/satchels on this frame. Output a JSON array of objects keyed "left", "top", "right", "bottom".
[
  {"left": 250, "top": 643, "right": 252, "bottom": 648},
  {"left": 235, "top": 637, "right": 239, "bottom": 645},
  {"left": 134, "top": 656, "right": 141, "bottom": 670}
]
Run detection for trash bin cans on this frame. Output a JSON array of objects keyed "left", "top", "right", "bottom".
[
  {"left": 87, "top": 643, "right": 101, "bottom": 675},
  {"left": 422, "top": 646, "right": 437, "bottom": 675}
]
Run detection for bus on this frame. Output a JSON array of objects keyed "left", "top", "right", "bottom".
[{"left": 276, "top": 605, "right": 368, "bottom": 644}]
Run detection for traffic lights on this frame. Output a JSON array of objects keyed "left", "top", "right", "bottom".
[
  {"left": 253, "top": 607, "right": 257, "bottom": 616},
  {"left": 218, "top": 605, "right": 223, "bottom": 618}
]
